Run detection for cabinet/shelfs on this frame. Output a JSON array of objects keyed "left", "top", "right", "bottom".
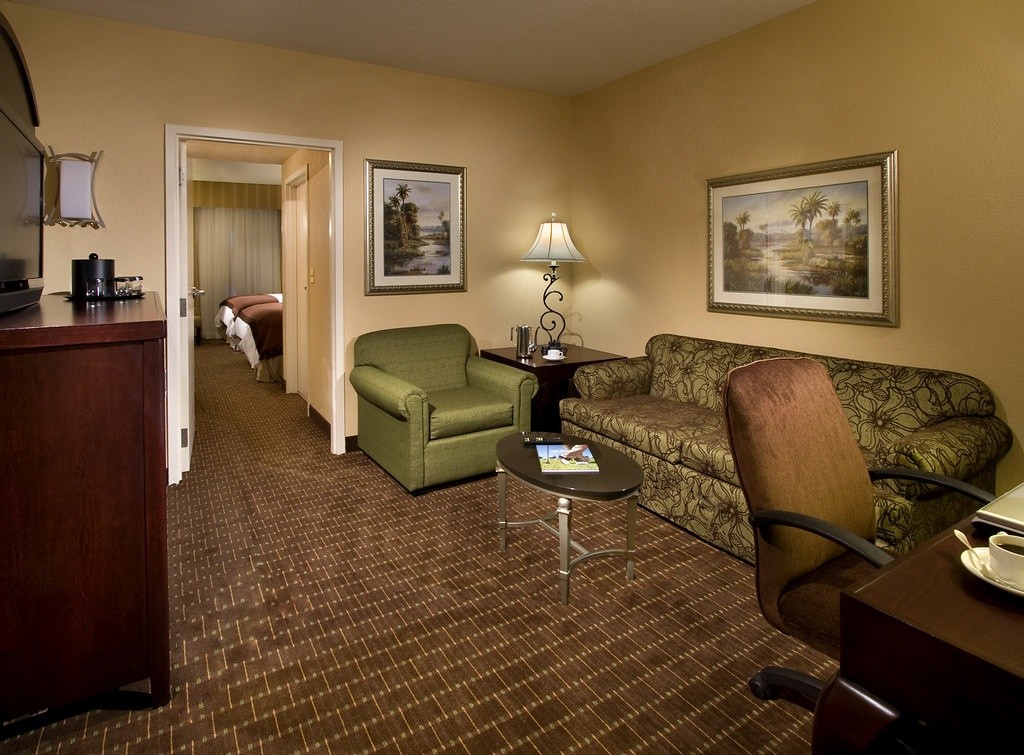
[{"left": 0, "top": 293, "right": 171, "bottom": 725}]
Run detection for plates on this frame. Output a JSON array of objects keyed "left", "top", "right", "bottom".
[
  {"left": 543, "top": 355, "right": 565, "bottom": 360},
  {"left": 960, "top": 547, "right": 1024, "bottom": 596}
]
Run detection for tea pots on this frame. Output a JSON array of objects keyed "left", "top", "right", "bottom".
[{"left": 510, "top": 323, "right": 540, "bottom": 359}]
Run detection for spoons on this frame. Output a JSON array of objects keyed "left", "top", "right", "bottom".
[{"left": 954, "top": 529, "right": 994, "bottom": 580}]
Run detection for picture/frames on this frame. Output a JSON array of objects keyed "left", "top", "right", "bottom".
[
  {"left": 705, "top": 149, "right": 900, "bottom": 328},
  {"left": 363, "top": 158, "right": 467, "bottom": 296}
]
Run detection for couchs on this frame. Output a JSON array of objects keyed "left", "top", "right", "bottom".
[
  {"left": 559, "top": 334, "right": 1013, "bottom": 567},
  {"left": 349, "top": 323, "right": 539, "bottom": 494}
]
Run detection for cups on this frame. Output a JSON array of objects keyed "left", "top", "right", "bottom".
[
  {"left": 114, "top": 278, "right": 129, "bottom": 295},
  {"left": 547, "top": 350, "right": 563, "bottom": 359},
  {"left": 989, "top": 532, "right": 1024, "bottom": 591},
  {"left": 85, "top": 277, "right": 104, "bottom": 297},
  {"left": 128, "top": 277, "right": 143, "bottom": 295}
]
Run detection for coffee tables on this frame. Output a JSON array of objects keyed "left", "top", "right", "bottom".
[{"left": 495, "top": 432, "right": 644, "bottom": 604}]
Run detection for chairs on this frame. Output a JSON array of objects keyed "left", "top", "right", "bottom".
[{"left": 722, "top": 357, "right": 996, "bottom": 755}]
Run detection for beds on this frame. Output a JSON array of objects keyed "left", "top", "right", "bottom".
[{"left": 214, "top": 293, "right": 282, "bottom": 382}]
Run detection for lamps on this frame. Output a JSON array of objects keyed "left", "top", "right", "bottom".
[
  {"left": 518, "top": 211, "right": 588, "bottom": 354},
  {"left": 45, "top": 146, "right": 105, "bottom": 230}
]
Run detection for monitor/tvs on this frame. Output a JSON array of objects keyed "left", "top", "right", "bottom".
[{"left": 0, "top": 99, "right": 45, "bottom": 313}]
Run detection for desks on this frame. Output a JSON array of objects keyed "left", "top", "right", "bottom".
[
  {"left": 481, "top": 342, "right": 628, "bottom": 433},
  {"left": 812, "top": 512, "right": 1024, "bottom": 755}
]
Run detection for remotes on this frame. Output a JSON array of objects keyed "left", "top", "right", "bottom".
[{"left": 523, "top": 437, "right": 563, "bottom": 445}]
